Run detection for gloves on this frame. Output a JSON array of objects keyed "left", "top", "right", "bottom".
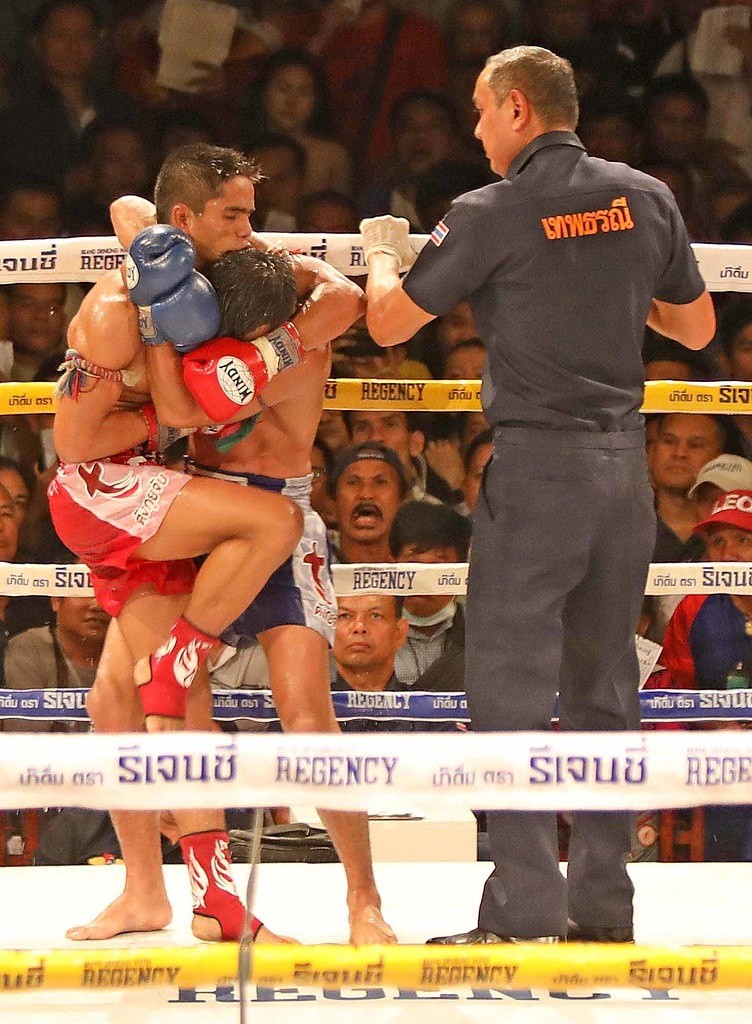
[
  {"left": 122, "top": 222, "right": 220, "bottom": 354},
  {"left": 181, "top": 324, "right": 306, "bottom": 424}
]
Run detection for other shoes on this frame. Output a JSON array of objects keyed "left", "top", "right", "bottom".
[
  {"left": 427, "top": 927, "right": 559, "bottom": 947},
  {"left": 229, "top": 822, "right": 341, "bottom": 863},
  {"left": 568, "top": 927, "right": 634, "bottom": 945}
]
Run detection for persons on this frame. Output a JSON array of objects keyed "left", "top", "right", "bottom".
[
  {"left": 357, "top": 46, "right": 715, "bottom": 953},
  {"left": 48, "top": 146, "right": 363, "bottom": 944},
  {"left": 0, "top": 0, "right": 752, "bottom": 866},
  {"left": 109, "top": 197, "right": 398, "bottom": 942}
]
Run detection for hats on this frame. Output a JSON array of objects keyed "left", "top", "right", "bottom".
[
  {"left": 687, "top": 454, "right": 752, "bottom": 499},
  {"left": 325, "top": 440, "right": 409, "bottom": 501},
  {"left": 692, "top": 489, "right": 752, "bottom": 532}
]
